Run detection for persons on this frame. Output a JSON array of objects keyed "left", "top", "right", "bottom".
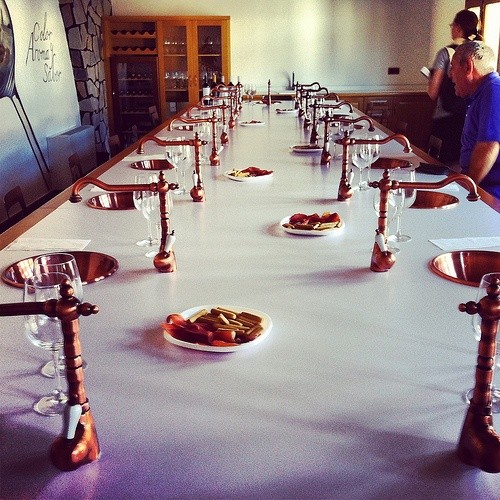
[
  {"left": 450, "top": 40, "right": 500, "bottom": 201},
  {"left": 426, "top": 9, "right": 485, "bottom": 164}
]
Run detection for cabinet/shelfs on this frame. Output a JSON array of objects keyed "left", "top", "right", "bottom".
[
  {"left": 340, "top": 91, "right": 432, "bottom": 146},
  {"left": 103, "top": 15, "right": 229, "bottom": 158}
]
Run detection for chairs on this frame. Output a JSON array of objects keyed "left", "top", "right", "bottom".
[
  {"left": 394, "top": 122, "right": 407, "bottom": 135},
  {"left": 4, "top": 184, "right": 27, "bottom": 225},
  {"left": 426, "top": 136, "right": 442, "bottom": 159},
  {"left": 365, "top": 103, "right": 373, "bottom": 118},
  {"left": 149, "top": 106, "right": 160, "bottom": 126},
  {"left": 109, "top": 135, "right": 121, "bottom": 157},
  {"left": 68, "top": 153, "right": 83, "bottom": 182}
]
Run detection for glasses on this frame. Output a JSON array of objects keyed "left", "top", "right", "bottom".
[{"left": 449, "top": 24, "right": 459, "bottom": 28}]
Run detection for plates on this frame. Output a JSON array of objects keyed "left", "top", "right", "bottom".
[
  {"left": 237, "top": 120, "right": 265, "bottom": 126},
  {"left": 223, "top": 168, "right": 275, "bottom": 182},
  {"left": 290, "top": 145, "right": 323, "bottom": 152},
  {"left": 275, "top": 108, "right": 298, "bottom": 114},
  {"left": 280, "top": 216, "right": 345, "bottom": 236},
  {"left": 162, "top": 305, "right": 273, "bottom": 353}
]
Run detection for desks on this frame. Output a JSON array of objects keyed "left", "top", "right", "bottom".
[{"left": 0, "top": 99, "right": 500, "bottom": 500}]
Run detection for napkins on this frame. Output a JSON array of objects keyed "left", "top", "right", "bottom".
[{"left": 7, "top": 114, "right": 500, "bottom": 283}]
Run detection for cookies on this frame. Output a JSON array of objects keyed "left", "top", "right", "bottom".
[
  {"left": 187, "top": 307, "right": 263, "bottom": 339},
  {"left": 229, "top": 168, "right": 250, "bottom": 177},
  {"left": 282, "top": 221, "right": 340, "bottom": 230}
]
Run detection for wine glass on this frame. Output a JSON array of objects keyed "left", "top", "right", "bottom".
[
  {"left": 133, "top": 87, "right": 240, "bottom": 258},
  {"left": 22, "top": 253, "right": 89, "bottom": 416},
  {"left": 295, "top": 84, "right": 416, "bottom": 257},
  {"left": 466, "top": 270, "right": 500, "bottom": 414},
  {"left": 243, "top": 83, "right": 256, "bottom": 104}
]
